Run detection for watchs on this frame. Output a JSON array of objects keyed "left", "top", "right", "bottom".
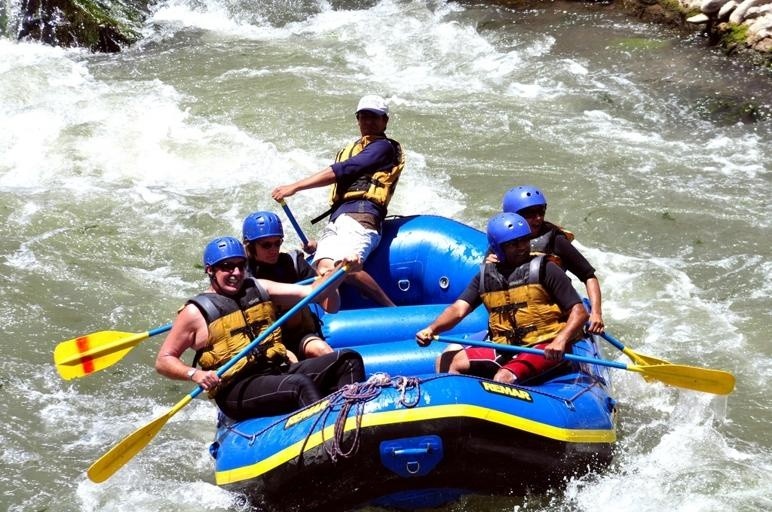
[{"left": 188, "top": 368, "right": 196, "bottom": 382}]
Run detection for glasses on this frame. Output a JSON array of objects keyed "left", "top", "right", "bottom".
[
  {"left": 255, "top": 238, "right": 283, "bottom": 249},
  {"left": 218, "top": 261, "right": 245, "bottom": 271},
  {"left": 518, "top": 206, "right": 545, "bottom": 218}
]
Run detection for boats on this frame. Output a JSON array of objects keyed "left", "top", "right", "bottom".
[{"left": 208, "top": 211, "right": 618, "bottom": 511}]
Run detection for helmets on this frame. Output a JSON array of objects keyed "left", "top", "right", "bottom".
[
  {"left": 204, "top": 236, "right": 248, "bottom": 273},
  {"left": 487, "top": 212, "right": 532, "bottom": 266},
  {"left": 243, "top": 211, "right": 284, "bottom": 241},
  {"left": 502, "top": 185, "right": 547, "bottom": 213},
  {"left": 356, "top": 95, "right": 389, "bottom": 119}
]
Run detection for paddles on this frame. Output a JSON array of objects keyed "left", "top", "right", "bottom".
[
  {"left": 86, "top": 266, "right": 350, "bottom": 483},
  {"left": 433, "top": 335, "right": 735, "bottom": 394},
  {"left": 588, "top": 323, "right": 671, "bottom": 382},
  {"left": 53, "top": 275, "right": 324, "bottom": 381}
]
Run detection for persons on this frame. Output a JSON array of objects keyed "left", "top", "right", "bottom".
[
  {"left": 415, "top": 211, "right": 590, "bottom": 384},
  {"left": 435, "top": 186, "right": 605, "bottom": 353},
  {"left": 271, "top": 96, "right": 404, "bottom": 314},
  {"left": 155, "top": 239, "right": 366, "bottom": 423},
  {"left": 243, "top": 213, "right": 341, "bottom": 364}
]
